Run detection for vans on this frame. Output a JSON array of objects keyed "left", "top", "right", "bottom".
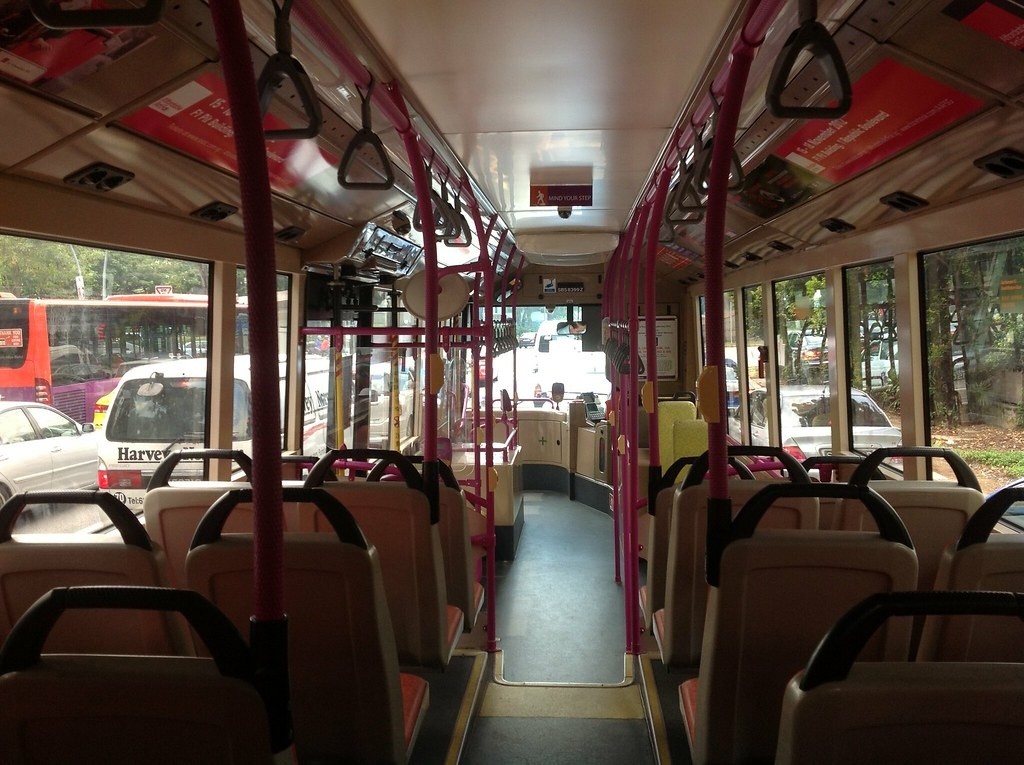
[
  {"left": 533, "top": 318, "right": 614, "bottom": 409},
  {"left": 98, "top": 354, "right": 344, "bottom": 510}
]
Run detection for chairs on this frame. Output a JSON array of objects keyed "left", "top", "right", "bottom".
[
  {"left": 0, "top": 586, "right": 270, "bottom": 765},
  {"left": 367, "top": 457, "right": 485, "bottom": 633},
  {"left": 831, "top": 447, "right": 985, "bottom": 661},
  {"left": 185, "top": 490, "right": 429, "bottom": 765},
  {"left": 652, "top": 446, "right": 820, "bottom": 665},
  {"left": 0, "top": 490, "right": 196, "bottom": 657},
  {"left": 298, "top": 448, "right": 463, "bottom": 672},
  {"left": 916, "top": 488, "right": 1024, "bottom": 663},
  {"left": 678, "top": 481, "right": 919, "bottom": 765},
  {"left": 639, "top": 456, "right": 756, "bottom": 630},
  {"left": 143, "top": 450, "right": 287, "bottom": 590},
  {"left": 777, "top": 590, "right": 1024, "bottom": 765}
]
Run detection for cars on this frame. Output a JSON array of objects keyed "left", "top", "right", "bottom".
[
  {"left": 788, "top": 330, "right": 829, "bottom": 362},
  {"left": 518, "top": 332, "right": 537, "bottom": 347},
  {"left": 725, "top": 360, "right": 765, "bottom": 411},
  {"left": 366, "top": 354, "right": 500, "bottom": 444},
  {"left": 0, "top": 403, "right": 102, "bottom": 517},
  {"left": 860, "top": 321, "right": 962, "bottom": 385},
  {"left": 728, "top": 384, "right": 903, "bottom": 465}
]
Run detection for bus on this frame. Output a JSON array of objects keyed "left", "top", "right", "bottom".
[{"left": 0, "top": 292, "right": 254, "bottom": 431}]
